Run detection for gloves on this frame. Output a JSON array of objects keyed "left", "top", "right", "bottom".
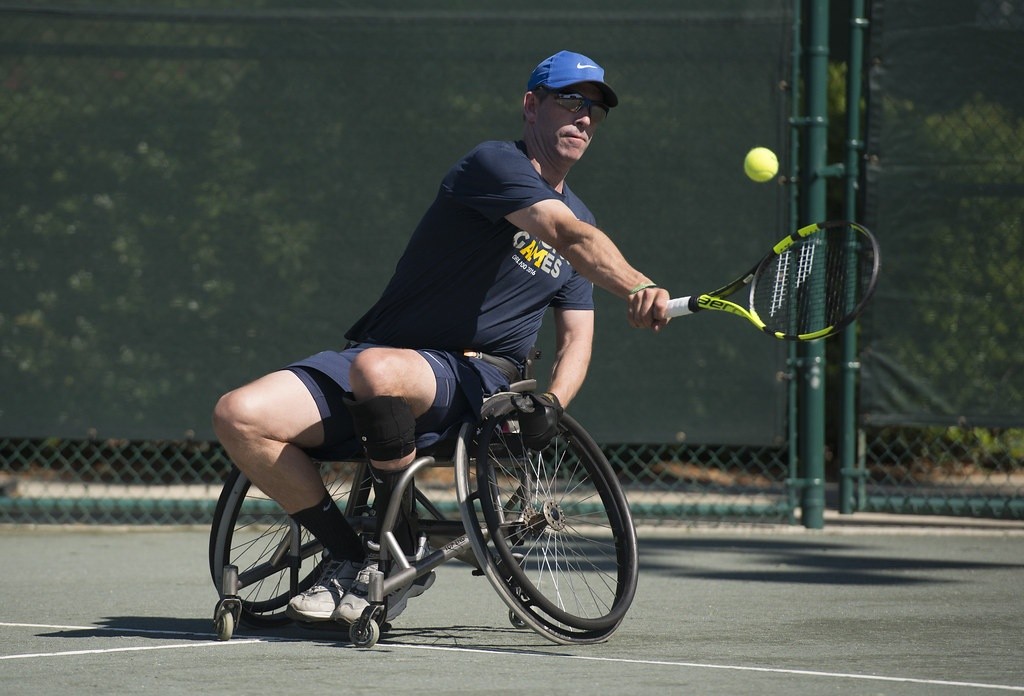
[{"left": 510, "top": 392, "right": 563, "bottom": 452}]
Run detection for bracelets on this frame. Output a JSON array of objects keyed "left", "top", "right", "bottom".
[{"left": 627, "top": 282, "right": 658, "bottom": 297}]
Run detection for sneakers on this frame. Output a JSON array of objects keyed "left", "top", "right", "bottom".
[{"left": 285, "top": 532, "right": 436, "bottom": 626}]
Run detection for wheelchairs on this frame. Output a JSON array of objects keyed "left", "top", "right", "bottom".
[{"left": 209, "top": 345, "right": 638, "bottom": 650}]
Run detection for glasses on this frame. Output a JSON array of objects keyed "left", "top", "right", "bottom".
[{"left": 554, "top": 87, "right": 609, "bottom": 123}]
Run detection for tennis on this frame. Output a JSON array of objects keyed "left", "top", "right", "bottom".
[{"left": 744, "top": 146, "right": 780, "bottom": 184}]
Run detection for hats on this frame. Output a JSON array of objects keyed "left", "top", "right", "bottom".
[{"left": 528, "top": 50, "right": 618, "bottom": 108}]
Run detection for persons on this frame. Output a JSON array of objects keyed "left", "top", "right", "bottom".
[{"left": 213, "top": 50, "right": 672, "bottom": 629}]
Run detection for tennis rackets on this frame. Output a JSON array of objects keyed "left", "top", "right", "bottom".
[{"left": 666, "top": 218, "right": 883, "bottom": 342}]
